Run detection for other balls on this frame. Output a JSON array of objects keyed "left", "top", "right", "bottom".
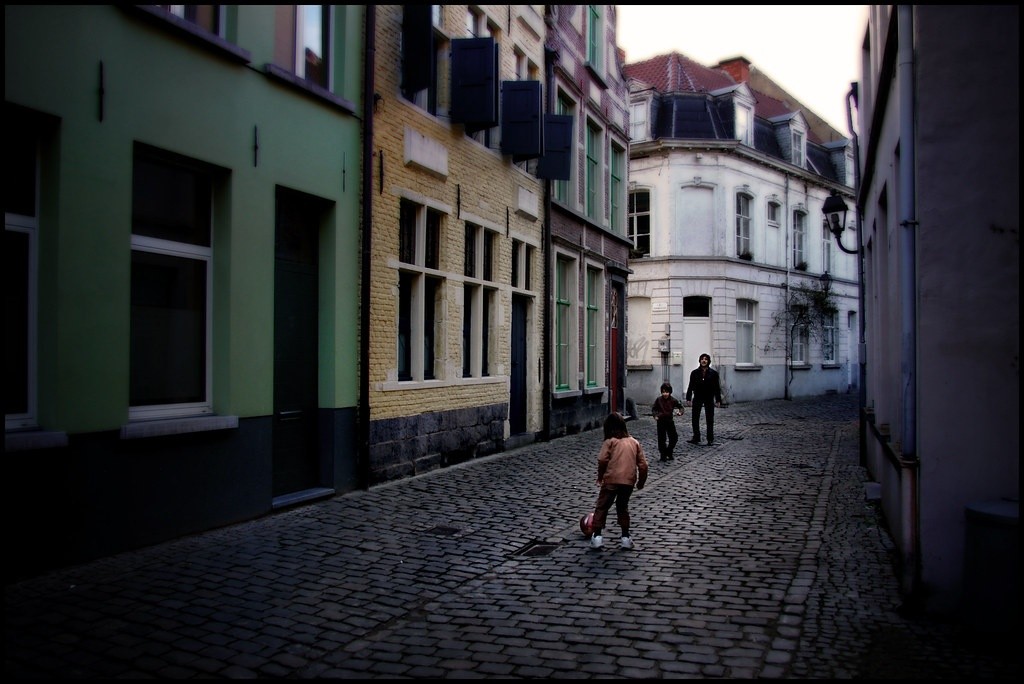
[{"left": 580, "top": 512, "right": 595, "bottom": 537}]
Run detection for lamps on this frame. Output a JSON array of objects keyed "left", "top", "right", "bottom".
[
  {"left": 819, "top": 270, "right": 834, "bottom": 302},
  {"left": 820, "top": 188, "right": 861, "bottom": 254}
]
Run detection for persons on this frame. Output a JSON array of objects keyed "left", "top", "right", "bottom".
[
  {"left": 686, "top": 353, "right": 723, "bottom": 447},
  {"left": 651, "top": 382, "right": 685, "bottom": 462},
  {"left": 588, "top": 413, "right": 648, "bottom": 549}
]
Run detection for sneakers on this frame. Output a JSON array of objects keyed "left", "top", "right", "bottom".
[
  {"left": 589, "top": 532, "right": 604, "bottom": 550},
  {"left": 621, "top": 536, "right": 634, "bottom": 550}
]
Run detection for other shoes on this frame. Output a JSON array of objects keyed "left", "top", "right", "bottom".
[
  {"left": 686, "top": 436, "right": 701, "bottom": 443},
  {"left": 707, "top": 439, "right": 713, "bottom": 446},
  {"left": 667, "top": 451, "right": 674, "bottom": 460},
  {"left": 662, "top": 453, "right": 667, "bottom": 462}
]
